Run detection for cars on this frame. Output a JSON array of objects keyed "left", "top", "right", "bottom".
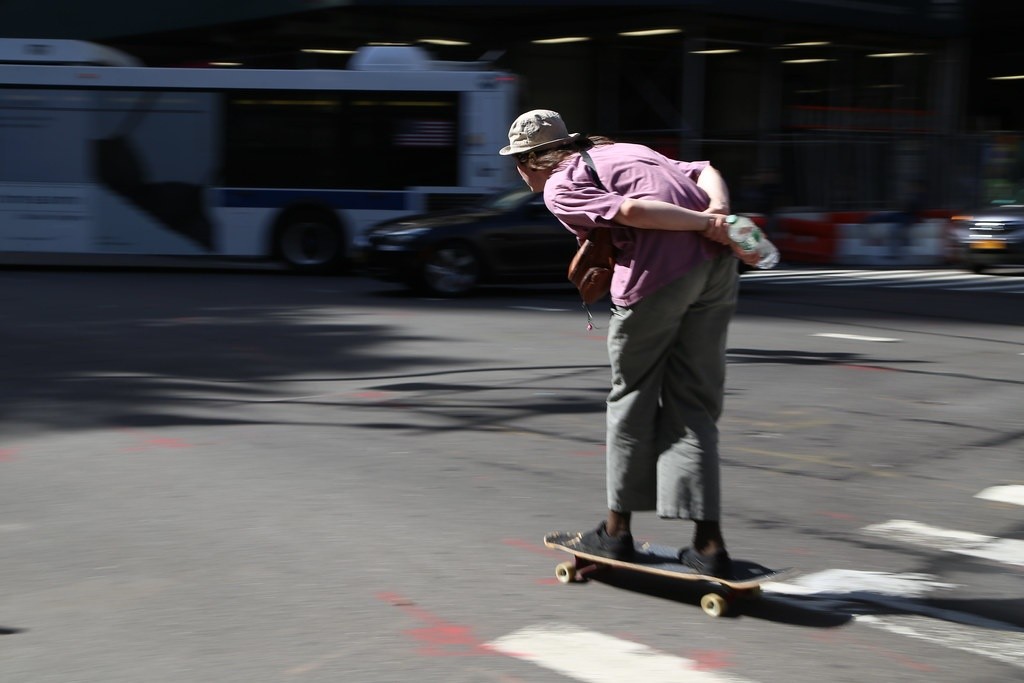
[
  {"left": 360, "top": 183, "right": 582, "bottom": 304},
  {"left": 950, "top": 202, "right": 1024, "bottom": 270}
]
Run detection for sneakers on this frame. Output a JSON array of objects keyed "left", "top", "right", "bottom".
[
  {"left": 579, "top": 520, "right": 635, "bottom": 559},
  {"left": 677, "top": 545, "right": 732, "bottom": 577}
]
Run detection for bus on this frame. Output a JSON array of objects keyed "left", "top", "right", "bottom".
[{"left": 0, "top": 37, "right": 516, "bottom": 270}]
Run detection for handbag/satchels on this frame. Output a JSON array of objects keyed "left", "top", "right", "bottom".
[{"left": 568, "top": 238, "right": 619, "bottom": 307}]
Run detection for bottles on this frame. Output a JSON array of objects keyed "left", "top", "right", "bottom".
[{"left": 725, "top": 214, "right": 780, "bottom": 269}]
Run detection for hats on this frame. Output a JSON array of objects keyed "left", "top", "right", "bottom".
[{"left": 499, "top": 109, "right": 580, "bottom": 155}]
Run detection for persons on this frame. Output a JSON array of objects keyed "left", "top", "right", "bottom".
[{"left": 498, "top": 109, "right": 759, "bottom": 575}]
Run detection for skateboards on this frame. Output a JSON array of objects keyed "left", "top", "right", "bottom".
[{"left": 544, "top": 529, "right": 796, "bottom": 616}]
